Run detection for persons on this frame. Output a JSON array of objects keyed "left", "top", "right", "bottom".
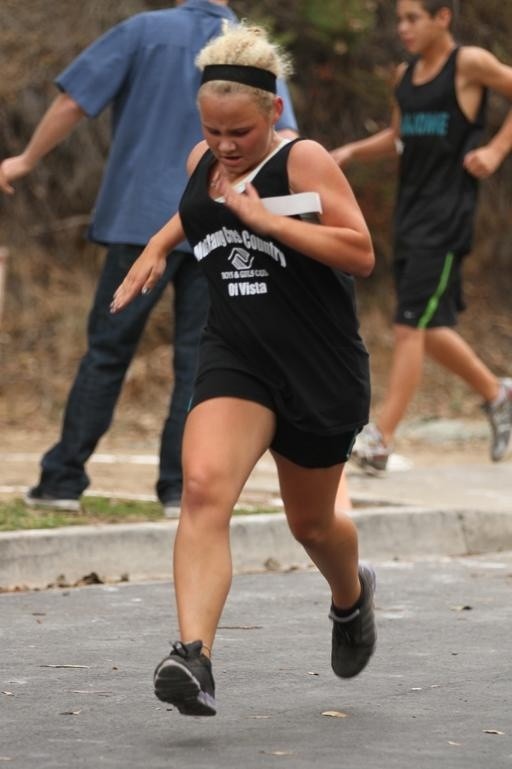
[
  {"left": 0, "top": 0, "right": 303, "bottom": 522},
  {"left": 323, "top": 1, "right": 510, "bottom": 481},
  {"left": 103, "top": 17, "right": 380, "bottom": 718}
]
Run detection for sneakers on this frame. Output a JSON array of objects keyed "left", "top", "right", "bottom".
[
  {"left": 482, "top": 377, "right": 512, "bottom": 460},
  {"left": 348, "top": 422, "right": 391, "bottom": 477},
  {"left": 329, "top": 563, "right": 375, "bottom": 677},
  {"left": 154, "top": 640, "right": 216, "bottom": 716},
  {"left": 24, "top": 488, "right": 80, "bottom": 511},
  {"left": 163, "top": 499, "right": 181, "bottom": 517}
]
